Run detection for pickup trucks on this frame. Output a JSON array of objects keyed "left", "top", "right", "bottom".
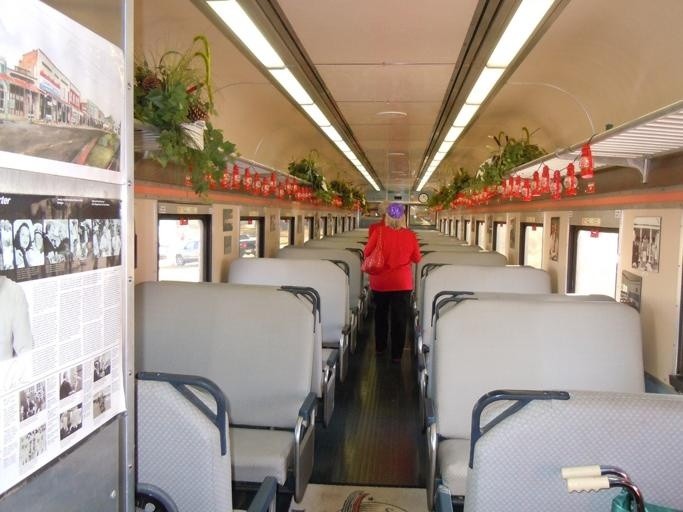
[{"left": 239, "top": 233, "right": 256, "bottom": 256}]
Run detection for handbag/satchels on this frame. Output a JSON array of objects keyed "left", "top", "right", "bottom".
[{"left": 360, "top": 224, "right": 385, "bottom": 275}]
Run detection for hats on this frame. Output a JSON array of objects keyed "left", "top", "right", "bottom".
[{"left": 385, "top": 203, "right": 403, "bottom": 219}]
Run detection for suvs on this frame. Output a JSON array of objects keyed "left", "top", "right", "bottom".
[{"left": 173, "top": 238, "right": 199, "bottom": 266}]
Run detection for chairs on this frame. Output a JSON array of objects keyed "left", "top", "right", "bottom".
[
  {"left": 409, "top": 227, "right": 682, "bottom": 512},
  {"left": 133, "top": 227, "right": 370, "bottom": 511}
]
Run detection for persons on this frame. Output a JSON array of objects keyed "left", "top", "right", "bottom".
[
  {"left": 0, "top": 216, "right": 123, "bottom": 276},
  {"left": 362, "top": 204, "right": 422, "bottom": 373},
  {"left": 1, "top": 274, "right": 35, "bottom": 359},
  {"left": 16, "top": 354, "right": 115, "bottom": 463}
]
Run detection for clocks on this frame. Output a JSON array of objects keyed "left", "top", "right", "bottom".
[{"left": 418, "top": 193, "right": 429, "bottom": 202}]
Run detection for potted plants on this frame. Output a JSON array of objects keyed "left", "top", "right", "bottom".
[{"left": 134, "top": 34, "right": 242, "bottom": 206}]
